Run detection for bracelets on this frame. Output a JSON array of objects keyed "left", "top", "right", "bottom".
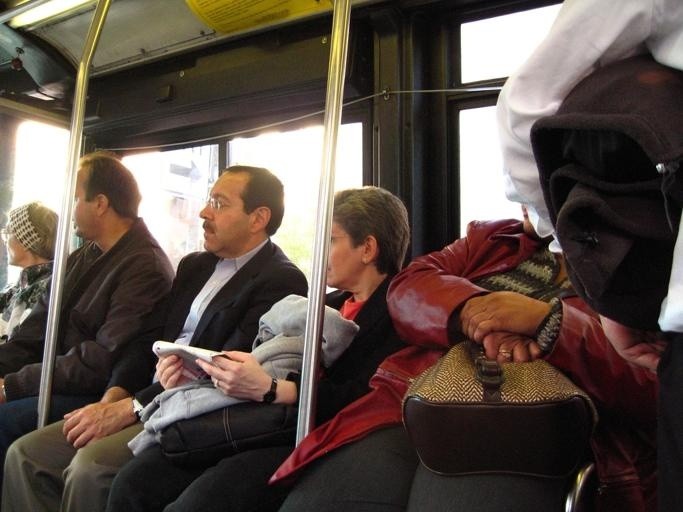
[
  {"left": 499, "top": 349, "right": 513, "bottom": 360},
  {"left": 131, "top": 397, "right": 145, "bottom": 421}
]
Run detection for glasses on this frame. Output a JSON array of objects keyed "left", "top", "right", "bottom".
[{"left": 204, "top": 196, "right": 230, "bottom": 218}]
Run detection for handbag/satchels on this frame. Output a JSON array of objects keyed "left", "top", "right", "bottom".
[
  {"left": 530, "top": 55, "right": 683, "bottom": 333},
  {"left": 399, "top": 339, "right": 603, "bottom": 484}
]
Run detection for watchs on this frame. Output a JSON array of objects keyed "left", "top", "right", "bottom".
[{"left": 263, "top": 378, "right": 278, "bottom": 403}]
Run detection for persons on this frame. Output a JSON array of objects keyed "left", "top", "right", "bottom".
[
  {"left": 495, "top": 0, "right": 683, "bottom": 510},
  {"left": 107, "top": 184, "right": 411, "bottom": 510},
  {"left": 265, "top": 201, "right": 660, "bottom": 509},
  {"left": 0, "top": 166, "right": 307, "bottom": 511},
  {"left": 0, "top": 152, "right": 177, "bottom": 497}
]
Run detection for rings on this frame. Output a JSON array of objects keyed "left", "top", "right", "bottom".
[{"left": 214, "top": 378, "right": 220, "bottom": 387}]
